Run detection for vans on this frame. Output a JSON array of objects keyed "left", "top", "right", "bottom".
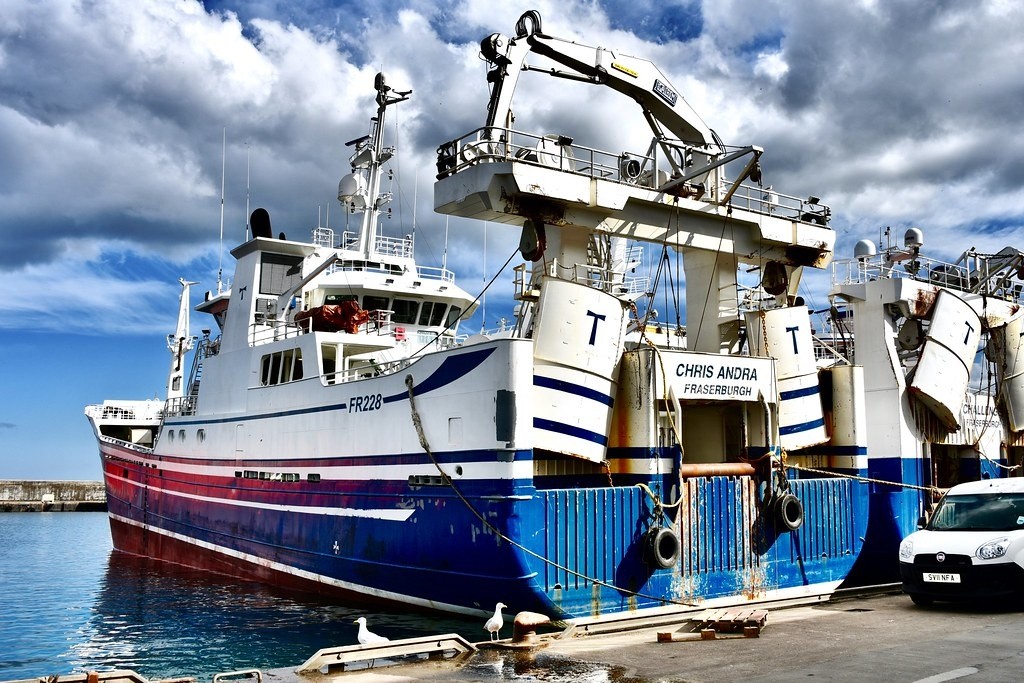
[{"left": 899, "top": 476, "right": 1024, "bottom": 612}]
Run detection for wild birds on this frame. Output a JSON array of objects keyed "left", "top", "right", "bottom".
[
  {"left": 354, "top": 617, "right": 390, "bottom": 668},
  {"left": 483, "top": 602, "right": 508, "bottom": 641}
]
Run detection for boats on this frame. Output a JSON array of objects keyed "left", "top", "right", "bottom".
[
  {"left": 84, "top": 10, "right": 869, "bottom": 633},
  {"left": 799, "top": 227, "right": 1024, "bottom": 582}
]
